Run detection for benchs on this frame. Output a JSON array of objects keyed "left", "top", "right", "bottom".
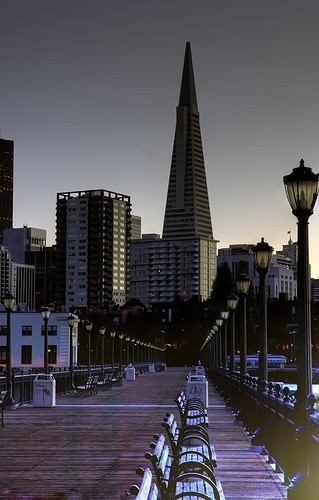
[
  {"left": 162, "top": 413, "right": 216, "bottom": 473},
  {"left": 144, "top": 433, "right": 217, "bottom": 500},
  {"left": 74, "top": 372, "right": 124, "bottom": 397},
  {"left": 130, "top": 467, "right": 226, "bottom": 500},
  {"left": 173, "top": 391, "right": 209, "bottom": 431}
]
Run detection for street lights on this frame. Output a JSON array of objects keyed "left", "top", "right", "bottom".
[
  {"left": 3, "top": 292, "right": 16, "bottom": 406},
  {"left": 66, "top": 314, "right": 76, "bottom": 390},
  {"left": 211, "top": 329, "right": 216, "bottom": 386},
  {"left": 84, "top": 321, "right": 94, "bottom": 377},
  {"left": 219, "top": 307, "right": 230, "bottom": 397},
  {"left": 136, "top": 339, "right": 140, "bottom": 374},
  {"left": 235, "top": 273, "right": 251, "bottom": 421},
  {"left": 144, "top": 342, "right": 147, "bottom": 373},
  {"left": 250, "top": 236, "right": 274, "bottom": 447},
  {"left": 215, "top": 318, "right": 224, "bottom": 391},
  {"left": 118, "top": 333, "right": 124, "bottom": 375},
  {"left": 200, "top": 335, "right": 211, "bottom": 383},
  {"left": 98, "top": 326, "right": 106, "bottom": 370},
  {"left": 225, "top": 293, "right": 239, "bottom": 407},
  {"left": 125, "top": 335, "right": 130, "bottom": 368},
  {"left": 131, "top": 337, "right": 135, "bottom": 366},
  {"left": 147, "top": 343, "right": 165, "bottom": 372},
  {"left": 110, "top": 330, "right": 116, "bottom": 373},
  {"left": 212, "top": 324, "right": 219, "bottom": 387},
  {"left": 209, "top": 332, "right": 213, "bottom": 383},
  {"left": 283, "top": 159, "right": 319, "bottom": 500},
  {"left": 41, "top": 307, "right": 51, "bottom": 380},
  {"left": 140, "top": 341, "right": 144, "bottom": 373}
]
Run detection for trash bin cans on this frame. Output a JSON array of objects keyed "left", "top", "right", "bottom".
[
  {"left": 195, "top": 366, "right": 206, "bottom": 375},
  {"left": 32, "top": 373, "right": 57, "bottom": 408},
  {"left": 125, "top": 366, "right": 136, "bottom": 382},
  {"left": 185, "top": 374, "right": 209, "bottom": 409}
]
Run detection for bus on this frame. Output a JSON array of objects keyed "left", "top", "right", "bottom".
[{"left": 227, "top": 353, "right": 287, "bottom": 368}]
